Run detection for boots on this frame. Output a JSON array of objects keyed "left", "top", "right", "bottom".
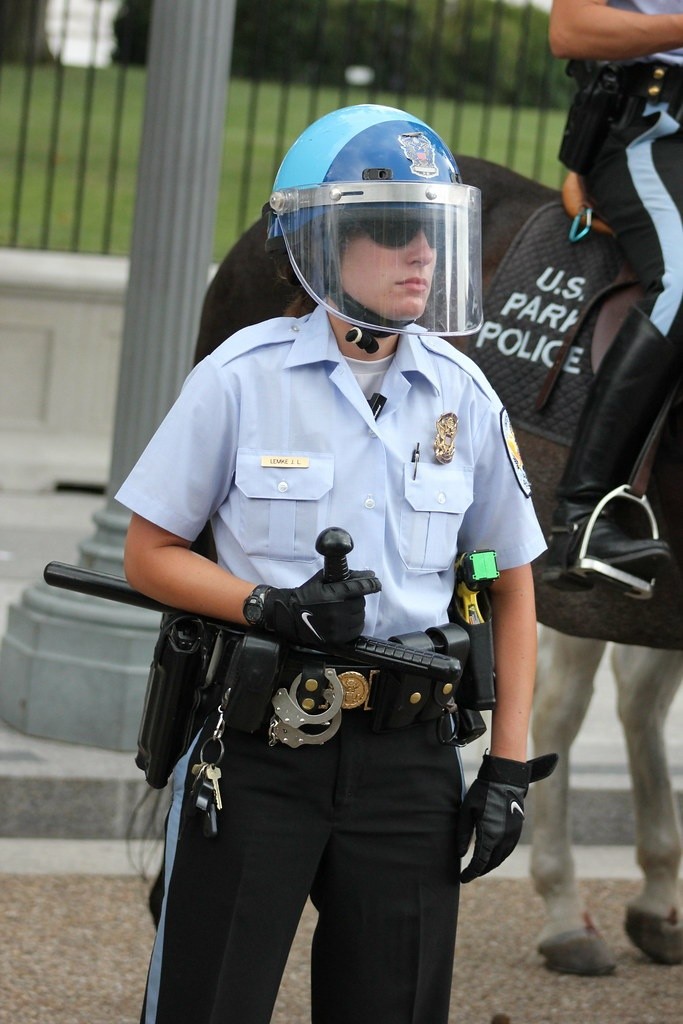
[{"left": 537, "top": 309, "right": 676, "bottom": 598}]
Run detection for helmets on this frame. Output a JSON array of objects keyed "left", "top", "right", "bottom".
[{"left": 269, "top": 104, "right": 470, "bottom": 235}]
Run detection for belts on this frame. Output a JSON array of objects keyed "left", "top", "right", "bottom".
[{"left": 273, "top": 650, "right": 385, "bottom": 727}]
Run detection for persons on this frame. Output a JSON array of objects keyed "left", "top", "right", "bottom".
[
  {"left": 540, "top": 0, "right": 682, "bottom": 593},
  {"left": 116, "top": 101, "right": 555, "bottom": 1024}
]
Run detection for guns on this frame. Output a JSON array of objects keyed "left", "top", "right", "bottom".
[
  {"left": 558, "top": 59, "right": 619, "bottom": 177},
  {"left": 135, "top": 612, "right": 199, "bottom": 788}
]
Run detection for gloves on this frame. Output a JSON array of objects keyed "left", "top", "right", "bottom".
[
  {"left": 246, "top": 562, "right": 381, "bottom": 647},
  {"left": 451, "top": 757, "right": 555, "bottom": 884}
]
